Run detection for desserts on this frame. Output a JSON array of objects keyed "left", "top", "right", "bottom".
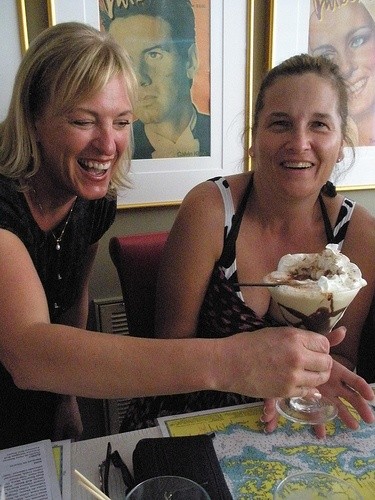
[{"left": 264, "top": 250, "right": 367, "bottom": 337}]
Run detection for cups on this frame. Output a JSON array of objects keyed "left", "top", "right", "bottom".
[
  {"left": 274, "top": 472, "right": 364, "bottom": 500},
  {"left": 124, "top": 475, "right": 211, "bottom": 500}
]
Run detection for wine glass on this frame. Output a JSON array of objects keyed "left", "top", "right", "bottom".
[{"left": 263, "top": 271, "right": 368, "bottom": 425}]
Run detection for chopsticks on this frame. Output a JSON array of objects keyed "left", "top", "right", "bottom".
[{"left": 73, "top": 469, "right": 112, "bottom": 500}]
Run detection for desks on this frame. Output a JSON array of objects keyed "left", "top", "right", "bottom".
[{"left": 0, "top": 382, "right": 374, "bottom": 499}]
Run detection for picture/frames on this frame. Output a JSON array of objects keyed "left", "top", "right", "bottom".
[{"left": 15, "top": 0, "right": 374, "bottom": 211}]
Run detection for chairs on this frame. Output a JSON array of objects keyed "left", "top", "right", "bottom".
[{"left": 108, "top": 231, "right": 174, "bottom": 432}]
